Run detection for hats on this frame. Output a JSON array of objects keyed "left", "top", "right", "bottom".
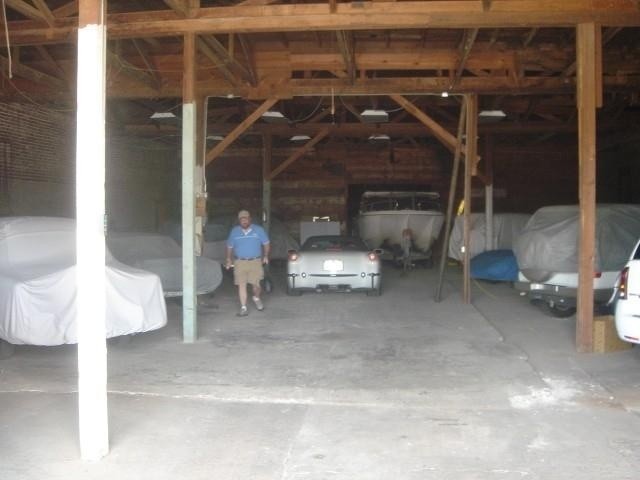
[{"left": 238, "top": 210, "right": 250, "bottom": 221}]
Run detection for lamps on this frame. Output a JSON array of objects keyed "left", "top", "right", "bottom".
[
  {"left": 261, "top": 109, "right": 284, "bottom": 119},
  {"left": 360, "top": 108, "right": 389, "bottom": 118},
  {"left": 369, "top": 132, "right": 390, "bottom": 140},
  {"left": 150, "top": 108, "right": 175, "bottom": 119},
  {"left": 207, "top": 134, "right": 224, "bottom": 141},
  {"left": 478, "top": 108, "right": 506, "bottom": 118},
  {"left": 291, "top": 132, "right": 311, "bottom": 141}
]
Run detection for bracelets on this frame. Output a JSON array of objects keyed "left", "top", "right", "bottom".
[
  {"left": 263, "top": 254, "right": 268, "bottom": 258},
  {"left": 226, "top": 258, "right": 232, "bottom": 262}
]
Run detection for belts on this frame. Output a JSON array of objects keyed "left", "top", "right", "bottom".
[{"left": 234, "top": 256, "right": 261, "bottom": 261}]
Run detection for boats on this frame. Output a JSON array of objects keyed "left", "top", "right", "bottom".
[{"left": 359, "top": 191, "right": 445, "bottom": 253}]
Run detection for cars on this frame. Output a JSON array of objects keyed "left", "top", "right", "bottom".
[{"left": 514, "top": 239, "right": 640, "bottom": 344}]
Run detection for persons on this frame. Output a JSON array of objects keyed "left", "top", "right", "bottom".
[{"left": 223, "top": 209, "right": 270, "bottom": 317}]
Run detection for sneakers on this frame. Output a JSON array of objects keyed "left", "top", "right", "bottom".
[
  {"left": 236, "top": 307, "right": 249, "bottom": 317},
  {"left": 252, "top": 295, "right": 264, "bottom": 311}
]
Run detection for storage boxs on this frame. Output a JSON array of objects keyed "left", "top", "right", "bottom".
[{"left": 594, "top": 315, "right": 633, "bottom": 353}]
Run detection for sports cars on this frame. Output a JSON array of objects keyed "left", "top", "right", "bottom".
[{"left": 287, "top": 236, "right": 384, "bottom": 295}]
